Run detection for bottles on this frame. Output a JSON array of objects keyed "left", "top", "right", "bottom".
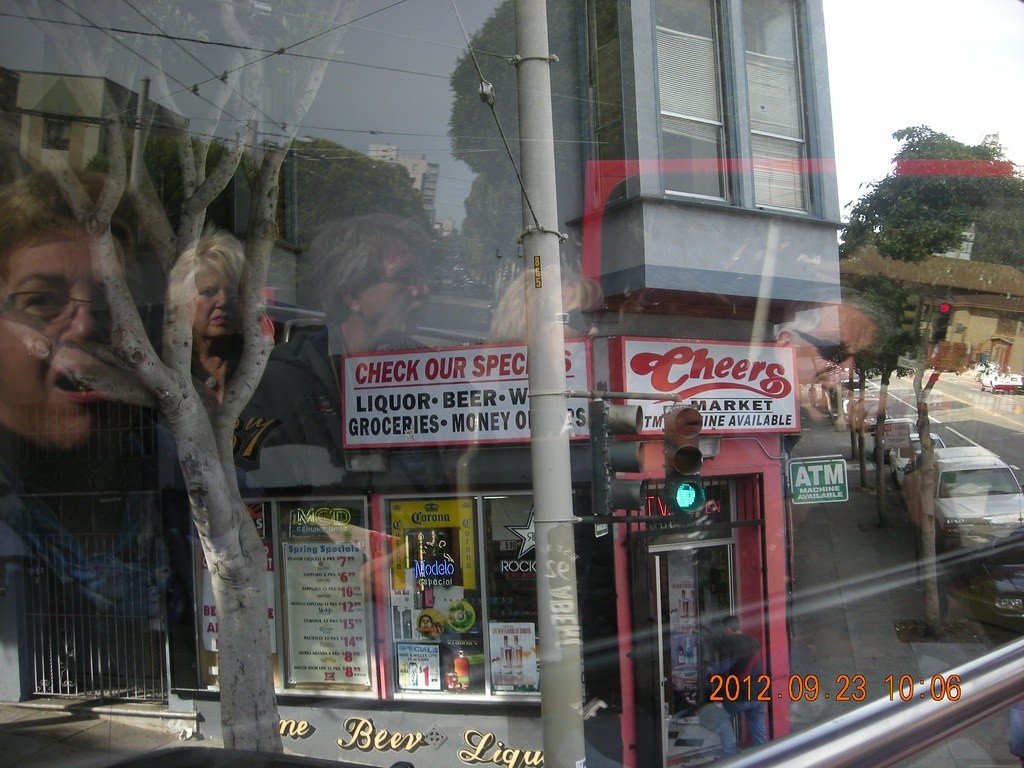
[
  {"left": 678, "top": 590, "right": 689, "bottom": 617},
  {"left": 400, "top": 664, "right": 408, "bottom": 686},
  {"left": 512, "top": 635, "right": 523, "bottom": 674},
  {"left": 500, "top": 636, "right": 512, "bottom": 674},
  {"left": 453, "top": 651, "right": 470, "bottom": 689},
  {"left": 687, "top": 590, "right": 696, "bottom": 616},
  {"left": 684, "top": 635, "right": 694, "bottom": 663},
  {"left": 677, "top": 644, "right": 686, "bottom": 665}
]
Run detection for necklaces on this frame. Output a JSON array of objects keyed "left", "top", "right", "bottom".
[{"left": 192, "top": 356, "right": 225, "bottom": 391}]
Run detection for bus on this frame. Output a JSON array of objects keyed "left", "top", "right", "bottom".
[{"left": 902, "top": 446, "right": 1024, "bottom": 551}]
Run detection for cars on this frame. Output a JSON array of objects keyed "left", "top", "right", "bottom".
[
  {"left": 975, "top": 361, "right": 1024, "bottom": 395},
  {"left": 952, "top": 512, "right": 1024, "bottom": 627},
  {"left": 830, "top": 375, "right": 890, "bottom": 432},
  {"left": 871, "top": 417, "right": 919, "bottom": 465},
  {"left": 889, "top": 432, "right": 947, "bottom": 493}
]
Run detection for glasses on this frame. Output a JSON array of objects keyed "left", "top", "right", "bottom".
[
  {"left": 373, "top": 267, "right": 424, "bottom": 286},
  {"left": 793, "top": 329, "right": 877, "bottom": 370},
  {"left": 565, "top": 308, "right": 619, "bottom": 332},
  {"left": 5, "top": 291, "right": 152, "bottom": 327}
]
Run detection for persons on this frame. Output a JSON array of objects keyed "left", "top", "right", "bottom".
[
  {"left": 0, "top": 163, "right": 895, "bottom": 475},
  {"left": 704, "top": 615, "right": 768, "bottom": 757},
  {"left": 806, "top": 384, "right": 819, "bottom": 409}
]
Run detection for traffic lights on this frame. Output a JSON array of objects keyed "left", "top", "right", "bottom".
[
  {"left": 589, "top": 400, "right": 647, "bottom": 516},
  {"left": 930, "top": 298, "right": 954, "bottom": 343},
  {"left": 664, "top": 404, "right": 706, "bottom": 515}
]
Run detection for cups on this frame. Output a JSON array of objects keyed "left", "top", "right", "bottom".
[{"left": 445, "top": 672, "right": 458, "bottom": 689}]
